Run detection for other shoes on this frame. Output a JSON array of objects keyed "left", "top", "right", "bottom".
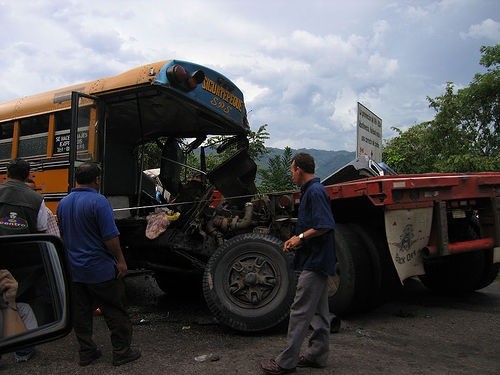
[{"left": 16, "top": 349, "right": 35, "bottom": 362}]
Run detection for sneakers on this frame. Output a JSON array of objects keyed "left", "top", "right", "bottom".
[
  {"left": 112, "top": 350, "right": 141, "bottom": 366},
  {"left": 79, "top": 350, "right": 101, "bottom": 366},
  {"left": 260, "top": 359, "right": 296, "bottom": 375},
  {"left": 296, "top": 352, "right": 325, "bottom": 368}
]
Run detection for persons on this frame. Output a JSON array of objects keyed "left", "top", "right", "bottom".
[
  {"left": 192, "top": 173, "right": 201, "bottom": 180},
  {"left": 260, "top": 153, "right": 339, "bottom": 375},
  {"left": 56, "top": 163, "right": 142, "bottom": 366},
  {"left": 0, "top": 270, "right": 38, "bottom": 342},
  {"left": 0, "top": 158, "right": 59, "bottom": 362}
]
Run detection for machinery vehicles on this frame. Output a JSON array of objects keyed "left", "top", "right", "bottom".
[{"left": 164, "top": 170, "right": 499, "bottom": 336}]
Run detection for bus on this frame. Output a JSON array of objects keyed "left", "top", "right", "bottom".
[{"left": 0, "top": 59, "right": 251, "bottom": 249}]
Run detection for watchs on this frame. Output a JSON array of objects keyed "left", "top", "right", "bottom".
[{"left": 299, "top": 233, "right": 304, "bottom": 240}]
